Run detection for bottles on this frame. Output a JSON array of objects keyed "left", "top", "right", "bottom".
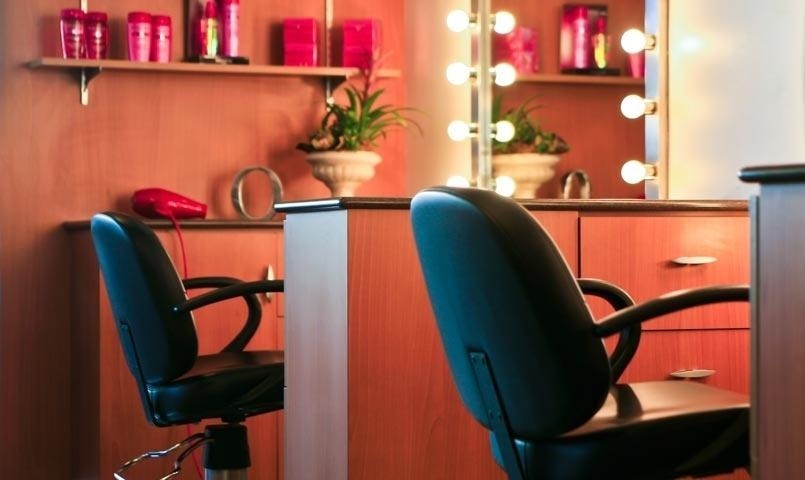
[
  {"left": 558, "top": 2, "right": 608, "bottom": 71},
  {"left": 58, "top": 0, "right": 242, "bottom": 67}
]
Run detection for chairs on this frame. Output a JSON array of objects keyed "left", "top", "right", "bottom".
[{"left": 396, "top": 186, "right": 753, "bottom": 479}]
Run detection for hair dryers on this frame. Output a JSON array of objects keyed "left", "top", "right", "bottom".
[{"left": 133, "top": 187, "right": 216, "bottom": 226}]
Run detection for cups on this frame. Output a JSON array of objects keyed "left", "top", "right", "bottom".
[
  {"left": 341, "top": 19, "right": 383, "bottom": 69},
  {"left": 280, "top": 16, "right": 321, "bottom": 67},
  {"left": 624, "top": 52, "right": 645, "bottom": 80},
  {"left": 490, "top": 26, "right": 542, "bottom": 77}
]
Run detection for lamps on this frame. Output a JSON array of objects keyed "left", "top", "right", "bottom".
[
  {"left": 615, "top": 30, "right": 664, "bottom": 186},
  {"left": 441, "top": 8, "right": 478, "bottom": 189},
  {"left": 488, "top": 12, "right": 526, "bottom": 199}
]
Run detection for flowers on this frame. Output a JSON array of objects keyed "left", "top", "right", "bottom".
[
  {"left": 492, "top": 35, "right": 568, "bottom": 152},
  {"left": 308, "top": 43, "right": 425, "bottom": 150}
]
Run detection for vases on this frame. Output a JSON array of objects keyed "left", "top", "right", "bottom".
[
  {"left": 303, "top": 147, "right": 386, "bottom": 200},
  {"left": 493, "top": 151, "right": 561, "bottom": 203}
]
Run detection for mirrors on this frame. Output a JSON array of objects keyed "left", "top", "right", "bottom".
[{"left": 476, "top": 0, "right": 670, "bottom": 201}]
